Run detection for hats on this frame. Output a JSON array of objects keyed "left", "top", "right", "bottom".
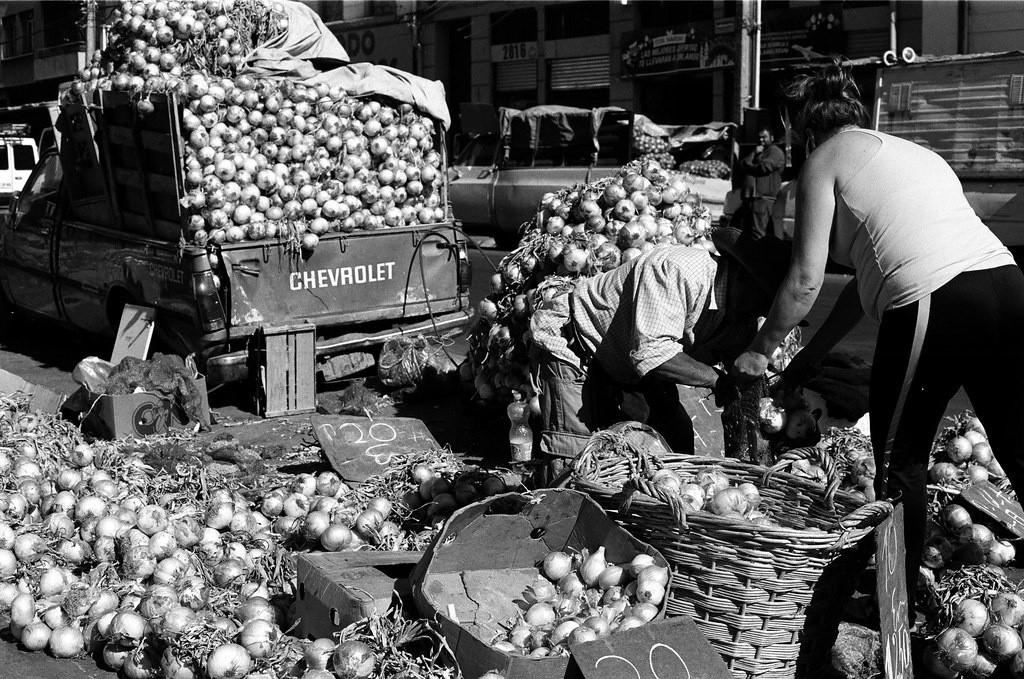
[{"left": 712, "top": 226, "right": 810, "bottom": 327}]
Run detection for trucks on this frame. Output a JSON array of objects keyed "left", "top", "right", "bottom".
[
  {"left": 723, "top": 51, "right": 1024, "bottom": 358},
  {"left": 0, "top": 86, "right": 473, "bottom": 380},
  {"left": 447, "top": 103, "right": 732, "bottom": 229}
]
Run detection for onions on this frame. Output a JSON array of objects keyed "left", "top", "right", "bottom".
[{"left": 1, "top": 0, "right": 1024, "bottom": 679}]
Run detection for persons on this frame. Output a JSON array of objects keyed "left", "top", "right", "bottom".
[
  {"left": 527, "top": 224, "right": 809, "bottom": 480},
  {"left": 744, "top": 128, "right": 785, "bottom": 238},
  {"left": 734, "top": 64, "right": 1024, "bottom": 630}
]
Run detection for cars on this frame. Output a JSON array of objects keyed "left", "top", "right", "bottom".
[{"left": 0, "top": 137, "right": 40, "bottom": 196}]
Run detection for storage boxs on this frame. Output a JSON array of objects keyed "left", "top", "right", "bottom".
[
  {"left": 83, "top": 372, "right": 210, "bottom": 441},
  {"left": 410, "top": 487, "right": 672, "bottom": 679},
  {"left": 293, "top": 551, "right": 425, "bottom": 645}
]
